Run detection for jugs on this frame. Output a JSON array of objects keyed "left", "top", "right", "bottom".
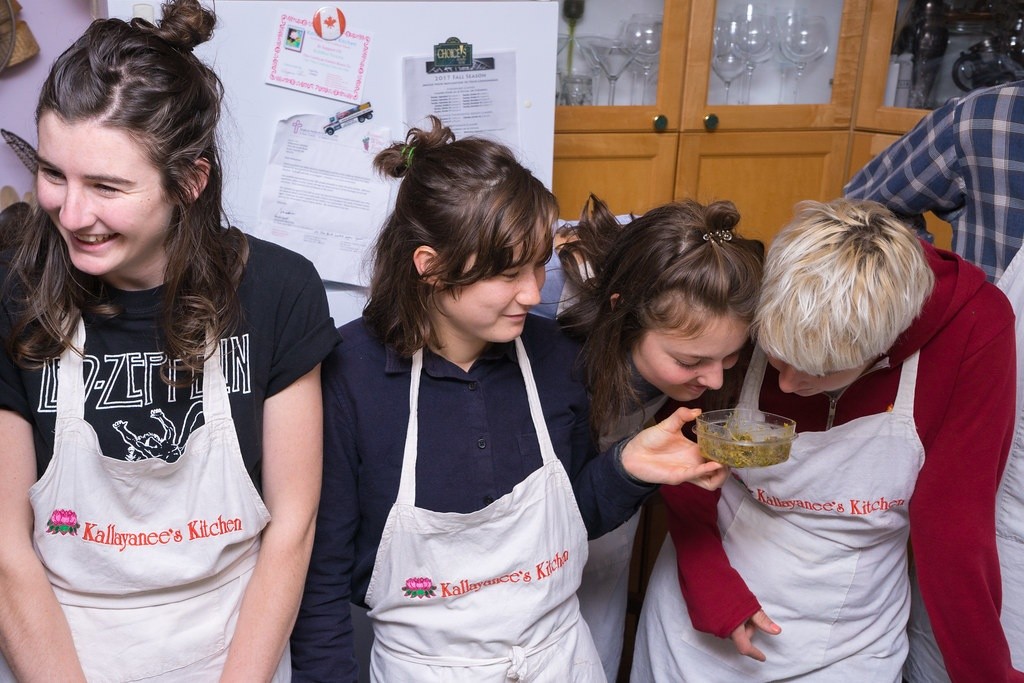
[{"left": 951, "top": 36, "right": 1017, "bottom": 90}]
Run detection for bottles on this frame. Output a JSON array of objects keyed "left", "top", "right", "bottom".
[{"left": 881, "top": 0, "right": 949, "bottom": 109}]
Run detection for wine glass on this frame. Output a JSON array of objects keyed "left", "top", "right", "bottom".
[
  {"left": 588, "top": 39, "right": 641, "bottom": 105},
  {"left": 712, "top": 52, "right": 744, "bottom": 104},
  {"left": 574, "top": 36, "right": 617, "bottom": 104},
  {"left": 618, "top": 21, "right": 647, "bottom": 104},
  {"left": 732, "top": 3, "right": 768, "bottom": 105},
  {"left": 730, "top": 16, "right": 778, "bottom": 104},
  {"left": 626, "top": 13, "right": 664, "bottom": 104},
  {"left": 780, "top": 15, "right": 829, "bottom": 104},
  {"left": 773, "top": 5, "right": 812, "bottom": 104}
]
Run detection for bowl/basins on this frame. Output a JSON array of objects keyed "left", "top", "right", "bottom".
[{"left": 688, "top": 409, "right": 798, "bottom": 467}]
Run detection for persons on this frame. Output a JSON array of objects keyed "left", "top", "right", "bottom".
[
  {"left": 628, "top": 200, "right": 1024, "bottom": 682},
  {"left": 1, "top": 0, "right": 343, "bottom": 683},
  {"left": 842, "top": 78, "right": 1023, "bottom": 282},
  {"left": 291, "top": 115, "right": 730, "bottom": 683},
  {"left": 521, "top": 192, "right": 766, "bottom": 683},
  {"left": 899, "top": 240, "right": 1023, "bottom": 683}
]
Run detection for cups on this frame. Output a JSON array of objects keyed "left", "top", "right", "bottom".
[
  {"left": 711, "top": 11, "right": 734, "bottom": 59},
  {"left": 560, "top": 76, "right": 594, "bottom": 105},
  {"left": 558, "top": 32, "right": 571, "bottom": 56}
]
